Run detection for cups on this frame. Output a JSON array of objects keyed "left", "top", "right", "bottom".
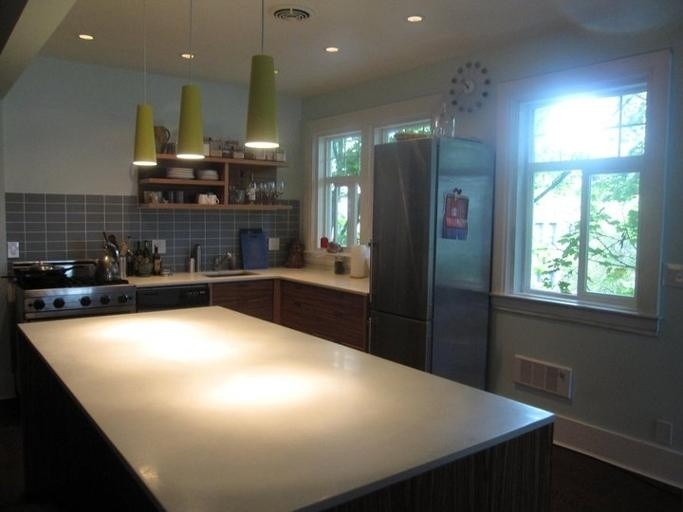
[
  {"left": 334, "top": 256, "right": 345, "bottom": 274},
  {"left": 186, "top": 257, "right": 196, "bottom": 273},
  {"left": 198, "top": 194, "right": 219, "bottom": 204},
  {"left": 141, "top": 189, "right": 186, "bottom": 204},
  {"left": 234, "top": 190, "right": 246, "bottom": 202},
  {"left": 321, "top": 237, "right": 328, "bottom": 248}
]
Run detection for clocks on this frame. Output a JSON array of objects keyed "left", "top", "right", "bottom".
[{"left": 448, "top": 60, "right": 490, "bottom": 115}]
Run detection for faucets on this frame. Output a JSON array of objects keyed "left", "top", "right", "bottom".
[{"left": 212, "top": 253, "right": 232, "bottom": 271}]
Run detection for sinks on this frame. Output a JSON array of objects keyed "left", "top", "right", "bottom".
[{"left": 205, "top": 271, "right": 260, "bottom": 278}]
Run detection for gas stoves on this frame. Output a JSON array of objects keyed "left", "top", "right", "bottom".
[{"left": 15, "top": 275, "right": 132, "bottom": 313}]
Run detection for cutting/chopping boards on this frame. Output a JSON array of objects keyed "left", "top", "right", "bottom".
[{"left": 240, "top": 229, "right": 269, "bottom": 269}]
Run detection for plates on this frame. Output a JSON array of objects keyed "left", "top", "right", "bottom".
[
  {"left": 196, "top": 168, "right": 219, "bottom": 180},
  {"left": 165, "top": 166, "right": 195, "bottom": 180},
  {"left": 394, "top": 133, "right": 425, "bottom": 139}
]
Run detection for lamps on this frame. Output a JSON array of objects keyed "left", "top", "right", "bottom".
[
  {"left": 245, "top": 0, "right": 280, "bottom": 150},
  {"left": 175, "top": 0, "right": 206, "bottom": 161},
  {"left": 133, "top": 0, "right": 158, "bottom": 165}
]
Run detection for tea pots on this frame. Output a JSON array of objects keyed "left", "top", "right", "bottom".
[
  {"left": 153, "top": 125, "right": 170, "bottom": 154},
  {"left": 97, "top": 241, "right": 120, "bottom": 281}
]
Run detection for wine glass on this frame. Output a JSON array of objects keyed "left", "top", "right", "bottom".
[{"left": 256, "top": 176, "right": 287, "bottom": 200}]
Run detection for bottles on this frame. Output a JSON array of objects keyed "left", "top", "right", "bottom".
[
  {"left": 430, "top": 101, "right": 457, "bottom": 137},
  {"left": 134, "top": 239, "right": 160, "bottom": 275},
  {"left": 193, "top": 244, "right": 202, "bottom": 272},
  {"left": 247, "top": 173, "right": 256, "bottom": 200}
]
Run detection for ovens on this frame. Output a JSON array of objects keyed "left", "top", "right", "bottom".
[{"left": 22, "top": 304, "right": 136, "bottom": 323}]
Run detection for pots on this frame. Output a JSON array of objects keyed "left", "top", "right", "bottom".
[{"left": 13, "top": 265, "right": 88, "bottom": 282}]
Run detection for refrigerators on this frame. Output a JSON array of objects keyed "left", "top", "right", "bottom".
[{"left": 366, "top": 138, "right": 495, "bottom": 392}]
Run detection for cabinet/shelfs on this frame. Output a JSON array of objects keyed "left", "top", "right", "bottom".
[
  {"left": 211, "top": 279, "right": 280, "bottom": 325},
  {"left": 136, "top": 154, "right": 293, "bottom": 211},
  {"left": 279, "top": 279, "right": 368, "bottom": 350}
]
